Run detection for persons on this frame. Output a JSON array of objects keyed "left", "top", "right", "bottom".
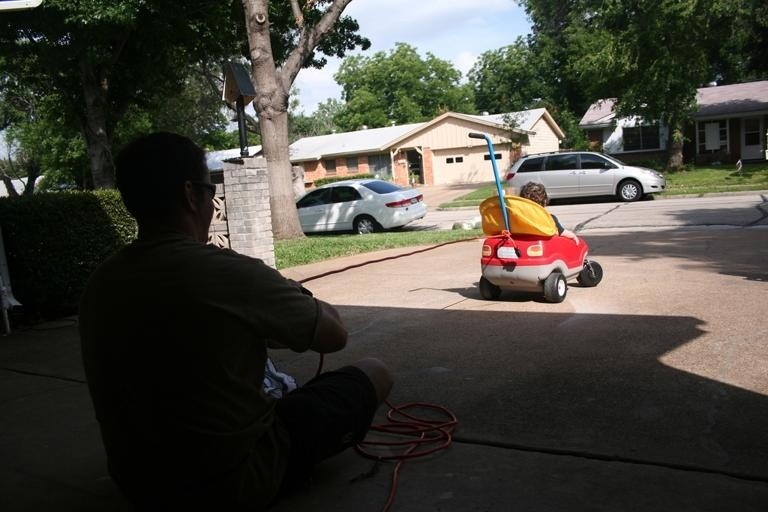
[
  {"left": 79, "top": 130, "right": 394, "bottom": 510},
  {"left": 519, "top": 182, "right": 578, "bottom": 242}
]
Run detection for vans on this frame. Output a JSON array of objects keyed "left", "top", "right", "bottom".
[{"left": 506, "top": 152, "right": 666, "bottom": 206}]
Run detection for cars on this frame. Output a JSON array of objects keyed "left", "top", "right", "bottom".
[{"left": 295, "top": 179, "right": 426, "bottom": 234}]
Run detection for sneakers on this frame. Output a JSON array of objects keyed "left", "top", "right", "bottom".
[{"left": 259, "top": 357, "right": 297, "bottom": 399}]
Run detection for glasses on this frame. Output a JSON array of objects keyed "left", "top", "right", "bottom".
[{"left": 193, "top": 182, "right": 216, "bottom": 201}]
